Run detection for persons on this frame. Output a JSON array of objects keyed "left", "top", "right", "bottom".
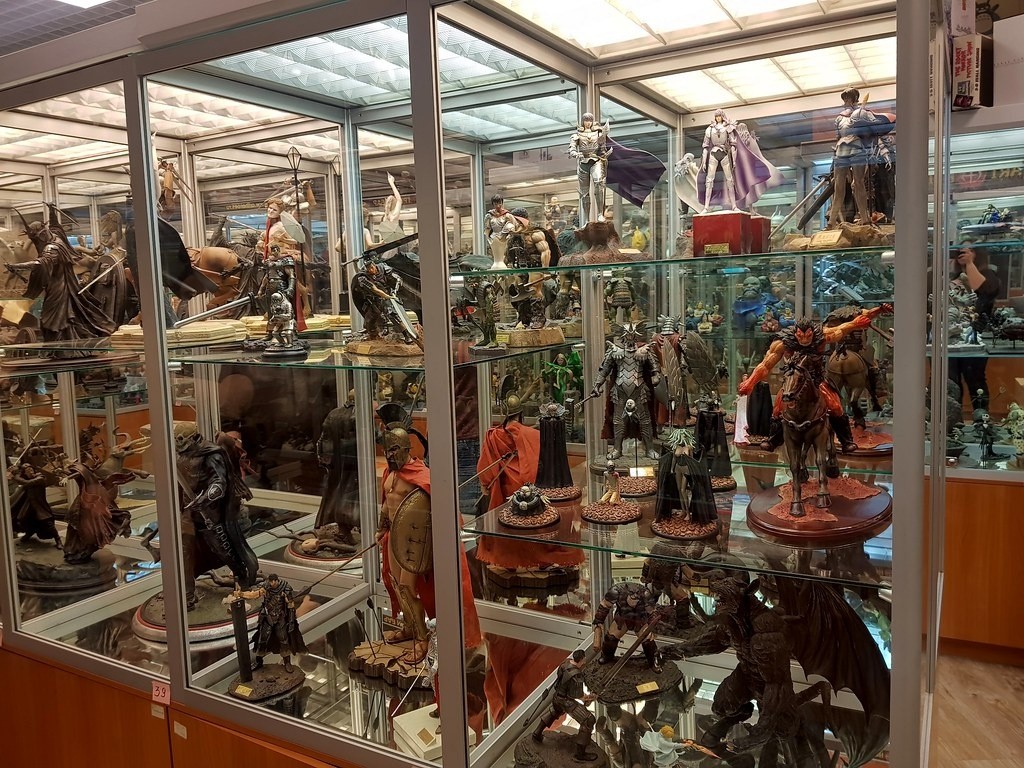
[
  {"left": 0, "top": 89, "right": 1024, "bottom": 761},
  {"left": 942, "top": 238, "right": 998, "bottom": 423}
]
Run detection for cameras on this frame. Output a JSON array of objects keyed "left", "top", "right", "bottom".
[{"left": 949, "top": 248, "right": 965, "bottom": 259}]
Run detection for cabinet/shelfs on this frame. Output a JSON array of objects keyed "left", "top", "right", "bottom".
[{"left": 0, "top": 0, "right": 1024, "bottom": 768}]
